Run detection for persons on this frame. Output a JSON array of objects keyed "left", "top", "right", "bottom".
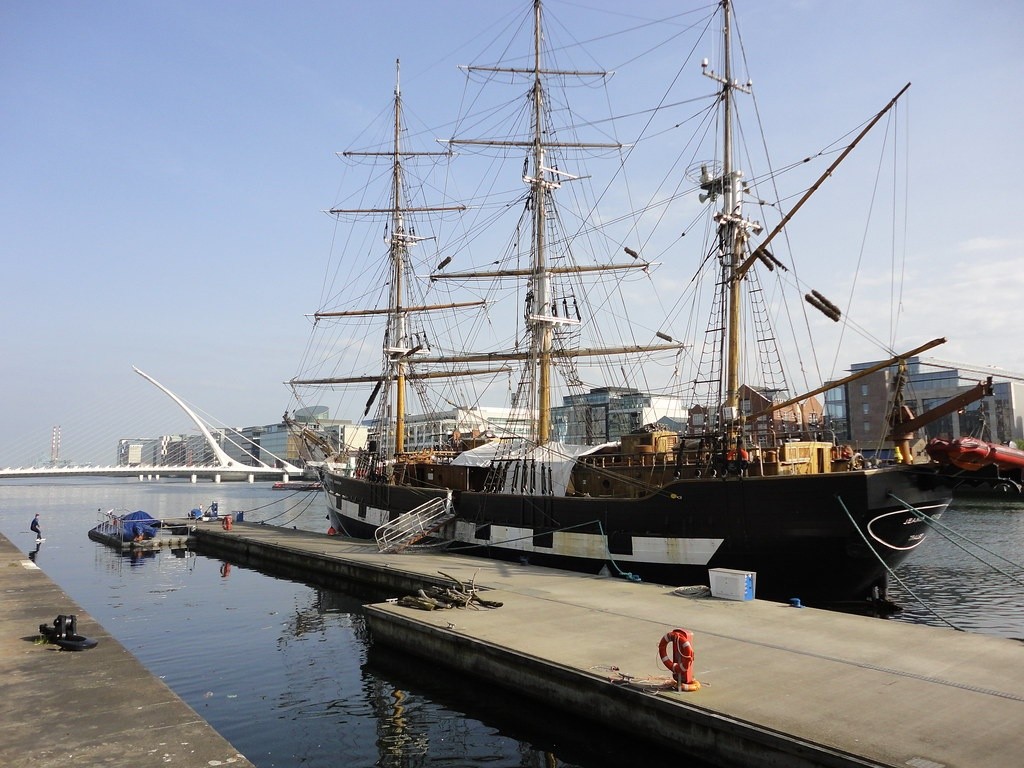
[{"left": 31, "top": 514, "right": 43, "bottom": 540}]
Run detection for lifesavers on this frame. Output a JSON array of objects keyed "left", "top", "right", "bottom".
[
  {"left": 222, "top": 516, "right": 232, "bottom": 530},
  {"left": 219, "top": 564, "right": 231, "bottom": 576},
  {"left": 667, "top": 676, "right": 702, "bottom": 691},
  {"left": 657, "top": 631, "right": 694, "bottom": 672}
]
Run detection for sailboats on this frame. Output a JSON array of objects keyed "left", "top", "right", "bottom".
[{"left": 281, "top": 0, "right": 994, "bottom": 617}]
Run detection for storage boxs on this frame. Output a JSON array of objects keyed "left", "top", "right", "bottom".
[{"left": 708, "top": 568, "right": 756, "bottom": 601}]
